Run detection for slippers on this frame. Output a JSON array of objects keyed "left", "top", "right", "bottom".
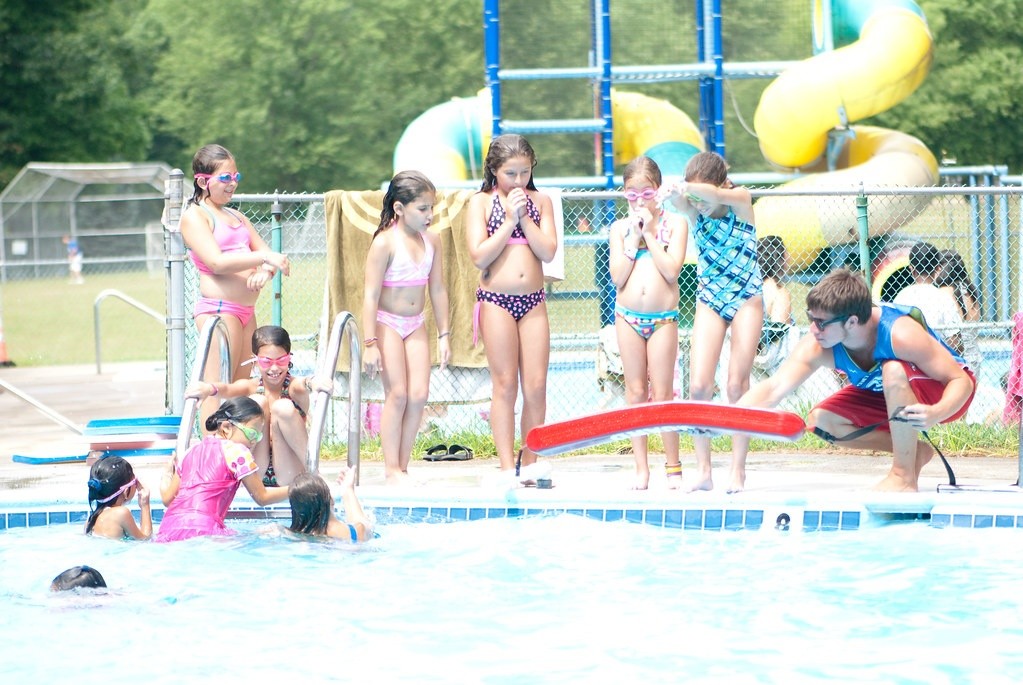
[
  {"left": 423, "top": 443, "right": 450, "bottom": 462},
  {"left": 441, "top": 444, "right": 475, "bottom": 461}
]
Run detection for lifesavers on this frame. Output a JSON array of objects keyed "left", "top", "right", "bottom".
[{"left": 526, "top": 400, "right": 807, "bottom": 458}]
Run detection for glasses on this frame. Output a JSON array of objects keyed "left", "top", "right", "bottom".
[
  {"left": 803, "top": 309, "right": 850, "bottom": 333},
  {"left": 252, "top": 351, "right": 292, "bottom": 369},
  {"left": 622, "top": 188, "right": 660, "bottom": 201},
  {"left": 192, "top": 173, "right": 243, "bottom": 184},
  {"left": 226, "top": 419, "right": 263, "bottom": 444}
]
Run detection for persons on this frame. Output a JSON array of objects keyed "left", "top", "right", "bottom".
[
  {"left": 608, "top": 154, "right": 688, "bottom": 493},
  {"left": 465, "top": 134, "right": 557, "bottom": 469},
  {"left": 757, "top": 235, "right": 795, "bottom": 355},
  {"left": 287, "top": 465, "right": 374, "bottom": 542},
  {"left": 735, "top": 266, "right": 976, "bottom": 489},
  {"left": 83, "top": 455, "right": 152, "bottom": 541},
  {"left": 49, "top": 564, "right": 108, "bottom": 592},
  {"left": 362, "top": 169, "right": 452, "bottom": 482},
  {"left": 893, "top": 242, "right": 981, "bottom": 353},
  {"left": 183, "top": 325, "right": 334, "bottom": 486},
  {"left": 156, "top": 397, "right": 289, "bottom": 544},
  {"left": 180, "top": 143, "right": 291, "bottom": 439},
  {"left": 656, "top": 151, "right": 764, "bottom": 494}
]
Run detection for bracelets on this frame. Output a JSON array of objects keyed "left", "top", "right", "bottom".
[
  {"left": 304, "top": 376, "right": 314, "bottom": 391},
  {"left": 520, "top": 213, "right": 527, "bottom": 218},
  {"left": 363, "top": 337, "right": 378, "bottom": 346},
  {"left": 259, "top": 268, "right": 275, "bottom": 280},
  {"left": 624, "top": 243, "right": 639, "bottom": 260},
  {"left": 265, "top": 254, "right": 267, "bottom": 263},
  {"left": 438, "top": 332, "right": 450, "bottom": 338},
  {"left": 211, "top": 382, "right": 218, "bottom": 396},
  {"left": 160, "top": 473, "right": 173, "bottom": 479}
]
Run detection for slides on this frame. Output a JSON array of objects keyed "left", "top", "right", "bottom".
[{"left": 393, "top": 0, "right": 983, "bottom": 321}]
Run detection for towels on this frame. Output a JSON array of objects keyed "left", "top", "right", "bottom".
[
  {"left": 1002, "top": 310, "right": 1022, "bottom": 433},
  {"left": 318, "top": 188, "right": 491, "bottom": 371}
]
